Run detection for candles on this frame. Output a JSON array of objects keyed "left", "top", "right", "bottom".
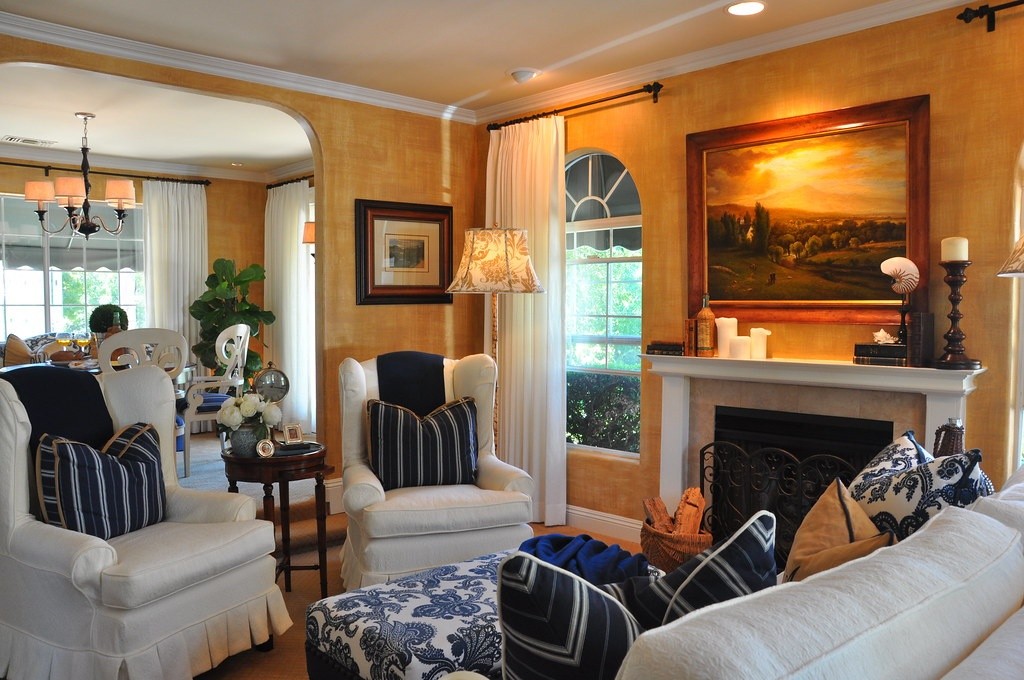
[
  {"left": 750, "top": 327, "right": 771, "bottom": 359},
  {"left": 714, "top": 317, "right": 738, "bottom": 357},
  {"left": 731, "top": 335, "right": 750, "bottom": 359}
]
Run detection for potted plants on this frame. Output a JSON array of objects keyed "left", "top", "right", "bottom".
[
  {"left": 89, "top": 304, "right": 128, "bottom": 350},
  {"left": 190, "top": 258, "right": 276, "bottom": 439}
]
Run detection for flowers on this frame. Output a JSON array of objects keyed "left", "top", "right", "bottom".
[{"left": 215, "top": 393, "right": 282, "bottom": 440}]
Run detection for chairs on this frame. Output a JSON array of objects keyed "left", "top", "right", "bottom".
[{"left": 98, "top": 328, "right": 188, "bottom": 479}]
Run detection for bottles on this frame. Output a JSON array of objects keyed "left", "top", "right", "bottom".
[
  {"left": 697, "top": 294, "right": 715, "bottom": 357},
  {"left": 105, "top": 312, "right": 129, "bottom": 370}
]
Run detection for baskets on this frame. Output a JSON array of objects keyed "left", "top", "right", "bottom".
[{"left": 640, "top": 518, "right": 713, "bottom": 574}]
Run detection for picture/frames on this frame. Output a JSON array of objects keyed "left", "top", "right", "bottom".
[
  {"left": 686, "top": 93, "right": 931, "bottom": 324},
  {"left": 283, "top": 422, "right": 303, "bottom": 445},
  {"left": 354, "top": 199, "right": 453, "bottom": 305}
]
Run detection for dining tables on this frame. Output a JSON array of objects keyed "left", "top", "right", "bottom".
[
  {"left": 0, "top": 359, "right": 196, "bottom": 377},
  {"left": 180, "top": 324, "right": 250, "bottom": 478}
]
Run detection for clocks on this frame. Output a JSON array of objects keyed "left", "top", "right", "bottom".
[
  {"left": 253, "top": 360, "right": 290, "bottom": 448},
  {"left": 940, "top": 237, "right": 969, "bottom": 261}
]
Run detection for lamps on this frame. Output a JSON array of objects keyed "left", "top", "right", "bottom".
[
  {"left": 25, "top": 111, "right": 137, "bottom": 240},
  {"left": 997, "top": 234, "right": 1024, "bottom": 278},
  {"left": 302, "top": 222, "right": 316, "bottom": 258},
  {"left": 445, "top": 225, "right": 547, "bottom": 454}
]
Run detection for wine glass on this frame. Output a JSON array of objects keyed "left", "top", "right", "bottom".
[
  {"left": 74, "top": 332, "right": 91, "bottom": 356},
  {"left": 56, "top": 332, "right": 72, "bottom": 352}
]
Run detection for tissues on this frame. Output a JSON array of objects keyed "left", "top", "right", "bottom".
[
  {"left": 730, "top": 336, "right": 751, "bottom": 358},
  {"left": 750, "top": 327, "right": 767, "bottom": 359},
  {"left": 715, "top": 316, "right": 738, "bottom": 357}
]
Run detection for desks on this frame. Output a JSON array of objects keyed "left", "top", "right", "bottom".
[{"left": 220, "top": 441, "right": 328, "bottom": 598}]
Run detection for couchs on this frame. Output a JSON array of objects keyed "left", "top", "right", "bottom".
[
  {"left": 0, "top": 333, "right": 89, "bottom": 368},
  {"left": 338, "top": 352, "right": 535, "bottom": 591},
  {"left": 442, "top": 464, "right": 1024, "bottom": 679},
  {"left": 0, "top": 365, "right": 292, "bottom": 680},
  {"left": 306, "top": 546, "right": 521, "bottom": 680}
]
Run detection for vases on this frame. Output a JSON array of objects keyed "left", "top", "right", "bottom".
[{"left": 231, "top": 423, "right": 266, "bottom": 455}]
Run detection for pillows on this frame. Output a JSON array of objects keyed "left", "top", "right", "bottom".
[
  {"left": 36, "top": 422, "right": 168, "bottom": 540},
  {"left": 367, "top": 396, "right": 478, "bottom": 491},
  {"left": 495, "top": 429, "right": 995, "bottom": 680}
]
